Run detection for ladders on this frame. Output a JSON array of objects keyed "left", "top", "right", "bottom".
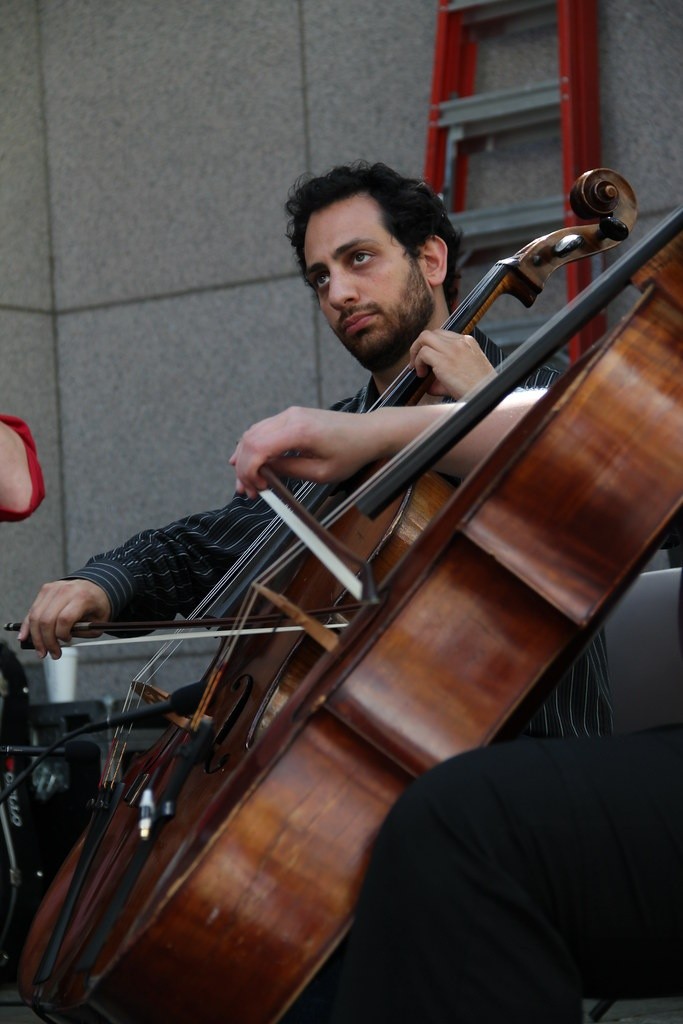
[{"left": 420, "top": 0, "right": 612, "bottom": 367}]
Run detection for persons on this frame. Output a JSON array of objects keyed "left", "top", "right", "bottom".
[
  {"left": 225, "top": 384, "right": 683, "bottom": 1024},
  {"left": 0, "top": 639, "right": 36, "bottom": 809},
  {"left": 17, "top": 157, "right": 614, "bottom": 1024}
]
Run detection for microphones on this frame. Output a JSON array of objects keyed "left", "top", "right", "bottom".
[{"left": 82, "top": 680, "right": 209, "bottom": 735}]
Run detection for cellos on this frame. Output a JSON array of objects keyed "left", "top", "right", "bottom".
[
  {"left": 16, "top": 167, "right": 639, "bottom": 1024},
  {"left": 81, "top": 243, "right": 683, "bottom": 1023}
]
